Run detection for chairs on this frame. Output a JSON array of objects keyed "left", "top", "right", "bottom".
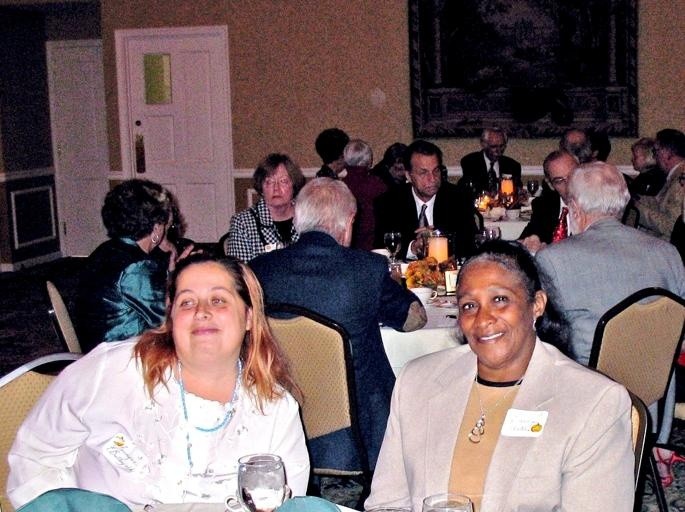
[
  {"left": 265, "top": 302, "right": 373, "bottom": 508},
  {"left": 585, "top": 286, "right": 685, "bottom": 508},
  {"left": 1, "top": 352, "right": 86, "bottom": 512},
  {"left": 36, "top": 277, "right": 89, "bottom": 365},
  {"left": 571, "top": 361, "right": 653, "bottom": 508}
]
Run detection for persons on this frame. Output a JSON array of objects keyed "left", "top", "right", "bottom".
[
  {"left": 561, "top": 127, "right": 592, "bottom": 163},
  {"left": 536, "top": 161, "right": 684, "bottom": 444},
  {"left": 365, "top": 238, "right": 635, "bottom": 511},
  {"left": 245, "top": 178, "right": 427, "bottom": 489},
  {"left": 629, "top": 127, "right": 684, "bottom": 240},
  {"left": 377, "top": 139, "right": 473, "bottom": 261},
  {"left": 7, "top": 253, "right": 310, "bottom": 511},
  {"left": 152, "top": 186, "right": 204, "bottom": 284},
  {"left": 461, "top": 127, "right": 523, "bottom": 196},
  {"left": 78, "top": 178, "right": 168, "bottom": 348},
  {"left": 314, "top": 128, "right": 349, "bottom": 185},
  {"left": 341, "top": 139, "right": 389, "bottom": 253},
  {"left": 517, "top": 150, "right": 583, "bottom": 251},
  {"left": 623, "top": 137, "right": 664, "bottom": 197},
  {"left": 225, "top": 154, "right": 303, "bottom": 263},
  {"left": 371, "top": 143, "right": 408, "bottom": 189}
]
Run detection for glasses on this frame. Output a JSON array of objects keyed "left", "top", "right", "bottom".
[{"left": 647, "top": 147, "right": 667, "bottom": 158}]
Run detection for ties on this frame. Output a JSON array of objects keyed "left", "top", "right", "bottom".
[
  {"left": 552, "top": 206, "right": 570, "bottom": 243},
  {"left": 485, "top": 162, "right": 497, "bottom": 190},
  {"left": 414, "top": 205, "right": 429, "bottom": 231}
]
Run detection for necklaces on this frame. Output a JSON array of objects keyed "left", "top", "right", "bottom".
[
  {"left": 178, "top": 359, "right": 242, "bottom": 466},
  {"left": 469, "top": 363, "right": 527, "bottom": 444}
]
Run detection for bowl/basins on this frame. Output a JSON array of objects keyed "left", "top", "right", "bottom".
[{"left": 506, "top": 210, "right": 520, "bottom": 218}]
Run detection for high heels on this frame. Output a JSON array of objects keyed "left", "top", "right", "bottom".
[{"left": 653, "top": 448, "right": 685, "bottom": 489}]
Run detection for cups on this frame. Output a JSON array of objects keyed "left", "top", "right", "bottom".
[
  {"left": 238, "top": 454, "right": 287, "bottom": 511},
  {"left": 421, "top": 491, "right": 473, "bottom": 512},
  {"left": 527, "top": 178, "right": 539, "bottom": 196},
  {"left": 444, "top": 269, "right": 458, "bottom": 291},
  {"left": 385, "top": 232, "right": 402, "bottom": 264},
  {"left": 409, "top": 287, "right": 437, "bottom": 304}
]
